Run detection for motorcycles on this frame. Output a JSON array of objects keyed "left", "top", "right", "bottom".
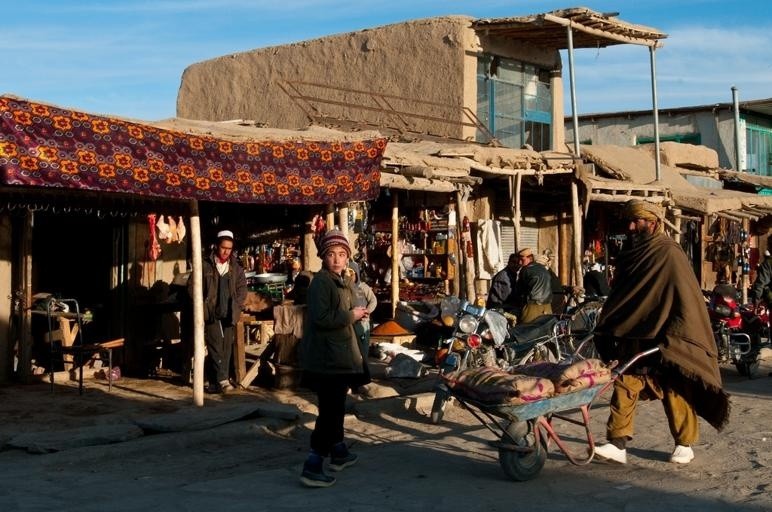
[
  {"left": 698, "top": 282, "right": 772, "bottom": 380},
  {"left": 426, "top": 253, "right": 570, "bottom": 425}
]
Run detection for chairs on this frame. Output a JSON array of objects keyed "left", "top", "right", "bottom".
[{"left": 46, "top": 297, "right": 125, "bottom": 395}]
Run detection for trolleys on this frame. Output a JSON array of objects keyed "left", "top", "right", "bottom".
[{"left": 443, "top": 331, "right": 663, "bottom": 484}]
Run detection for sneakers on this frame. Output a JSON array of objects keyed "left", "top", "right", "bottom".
[
  {"left": 670, "top": 445, "right": 695, "bottom": 464},
  {"left": 589, "top": 444, "right": 627, "bottom": 465},
  {"left": 208, "top": 381, "right": 223, "bottom": 393},
  {"left": 299, "top": 470, "right": 336, "bottom": 488},
  {"left": 218, "top": 379, "right": 233, "bottom": 394},
  {"left": 327, "top": 453, "right": 359, "bottom": 472}
]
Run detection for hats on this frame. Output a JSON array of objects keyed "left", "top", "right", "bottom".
[
  {"left": 319, "top": 229, "right": 351, "bottom": 258},
  {"left": 518, "top": 248, "right": 532, "bottom": 257},
  {"left": 218, "top": 231, "right": 233, "bottom": 239}
]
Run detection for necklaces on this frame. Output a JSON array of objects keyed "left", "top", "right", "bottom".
[{"left": 753, "top": 252, "right": 771, "bottom": 305}]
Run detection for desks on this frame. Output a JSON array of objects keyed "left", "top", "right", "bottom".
[{"left": 230, "top": 297, "right": 282, "bottom": 390}]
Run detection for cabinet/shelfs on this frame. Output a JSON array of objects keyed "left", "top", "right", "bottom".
[{"left": 396, "top": 222, "right": 458, "bottom": 280}]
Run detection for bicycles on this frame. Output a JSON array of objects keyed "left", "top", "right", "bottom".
[{"left": 548, "top": 283, "right": 607, "bottom": 361}]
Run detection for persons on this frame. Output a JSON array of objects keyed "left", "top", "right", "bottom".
[
  {"left": 301, "top": 229, "right": 370, "bottom": 487},
  {"left": 485, "top": 251, "right": 520, "bottom": 311},
  {"left": 581, "top": 249, "right": 609, "bottom": 298},
  {"left": 186, "top": 230, "right": 247, "bottom": 390},
  {"left": 516, "top": 247, "right": 561, "bottom": 323},
  {"left": 350, "top": 262, "right": 378, "bottom": 394},
  {"left": 592, "top": 199, "right": 733, "bottom": 461}
]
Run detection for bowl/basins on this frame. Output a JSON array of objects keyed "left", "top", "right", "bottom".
[{"left": 254, "top": 273, "right": 288, "bottom": 283}]
